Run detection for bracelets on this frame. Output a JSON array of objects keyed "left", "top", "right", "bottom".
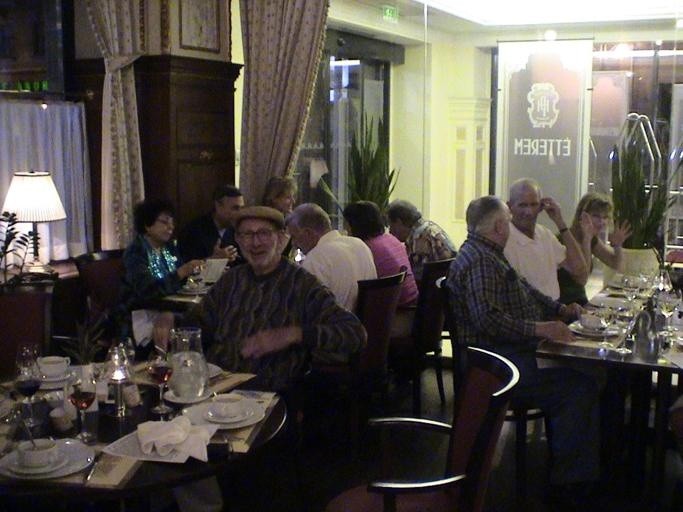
[{"left": 559, "top": 228, "right": 568, "bottom": 232}]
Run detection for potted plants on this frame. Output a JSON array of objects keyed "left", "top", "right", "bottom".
[{"left": 603, "top": 137, "right": 683, "bottom": 287}]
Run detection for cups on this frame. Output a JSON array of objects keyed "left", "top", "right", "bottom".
[
  {"left": 37, "top": 356, "right": 71, "bottom": 377},
  {"left": 579, "top": 315, "right": 602, "bottom": 331},
  {"left": 212, "top": 393, "right": 245, "bottom": 417},
  {"left": 167, "top": 326, "right": 208, "bottom": 400},
  {"left": 15, "top": 438, "right": 57, "bottom": 468}
]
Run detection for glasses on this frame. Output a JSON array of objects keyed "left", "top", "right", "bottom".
[
  {"left": 156, "top": 217, "right": 174, "bottom": 224},
  {"left": 236, "top": 228, "right": 274, "bottom": 238}
]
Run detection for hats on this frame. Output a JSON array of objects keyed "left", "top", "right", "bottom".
[{"left": 231, "top": 206, "right": 285, "bottom": 229}]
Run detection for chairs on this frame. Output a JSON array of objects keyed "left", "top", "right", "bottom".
[
  {"left": 320, "top": 266, "right": 407, "bottom": 463},
  {"left": 435, "top": 276, "right": 554, "bottom": 505},
  {"left": 389, "top": 258, "right": 456, "bottom": 417},
  {"left": 0, "top": 282, "right": 55, "bottom": 377},
  {"left": 69, "top": 250, "right": 126, "bottom": 313},
  {"left": 323, "top": 347, "right": 520, "bottom": 511}
]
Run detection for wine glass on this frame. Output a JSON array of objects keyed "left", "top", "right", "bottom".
[
  {"left": 188, "top": 261, "right": 207, "bottom": 303},
  {"left": 599, "top": 267, "right": 677, "bottom": 350},
  {"left": 17, "top": 342, "right": 42, "bottom": 405},
  {"left": 110, "top": 335, "right": 136, "bottom": 367},
  {"left": 68, "top": 374, "right": 97, "bottom": 442},
  {"left": 12, "top": 357, "right": 44, "bottom": 429},
  {"left": 146, "top": 353, "right": 173, "bottom": 414}
]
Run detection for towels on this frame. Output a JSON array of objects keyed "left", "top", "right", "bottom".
[{"left": 136, "top": 415, "right": 214, "bottom": 462}]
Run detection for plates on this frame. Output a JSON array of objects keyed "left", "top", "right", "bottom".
[
  {"left": 5, "top": 450, "right": 68, "bottom": 475},
  {"left": 35, "top": 373, "right": 71, "bottom": 383},
  {"left": 205, "top": 407, "right": 255, "bottom": 424},
  {"left": 182, "top": 398, "right": 264, "bottom": 430},
  {"left": 568, "top": 321, "right": 625, "bottom": 338},
  {"left": 0, "top": 438, "right": 96, "bottom": 481},
  {"left": 175, "top": 284, "right": 212, "bottom": 296},
  {"left": 163, "top": 388, "right": 212, "bottom": 404},
  {"left": 39, "top": 378, "right": 81, "bottom": 391},
  {"left": 208, "top": 362, "right": 223, "bottom": 380}
]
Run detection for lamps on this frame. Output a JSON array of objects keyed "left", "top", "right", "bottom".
[{"left": 0, "top": 171, "right": 68, "bottom": 275}]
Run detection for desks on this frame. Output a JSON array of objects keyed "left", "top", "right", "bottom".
[{"left": 0, "top": 263, "right": 81, "bottom": 336}]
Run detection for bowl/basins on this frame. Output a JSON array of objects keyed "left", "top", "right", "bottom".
[{"left": 44, "top": 390, "right": 64, "bottom": 408}]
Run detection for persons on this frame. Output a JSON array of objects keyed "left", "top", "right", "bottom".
[
  {"left": 110, "top": 198, "right": 208, "bottom": 363},
  {"left": 261, "top": 176, "right": 299, "bottom": 267},
  {"left": 501, "top": 181, "right": 587, "bottom": 304},
  {"left": 178, "top": 185, "right": 245, "bottom": 268},
  {"left": 342, "top": 199, "right": 420, "bottom": 371},
  {"left": 176, "top": 203, "right": 368, "bottom": 512},
  {"left": 555, "top": 192, "right": 634, "bottom": 307},
  {"left": 386, "top": 201, "right": 457, "bottom": 288},
  {"left": 442, "top": 195, "right": 608, "bottom": 486},
  {"left": 287, "top": 201, "right": 378, "bottom": 314}
]
